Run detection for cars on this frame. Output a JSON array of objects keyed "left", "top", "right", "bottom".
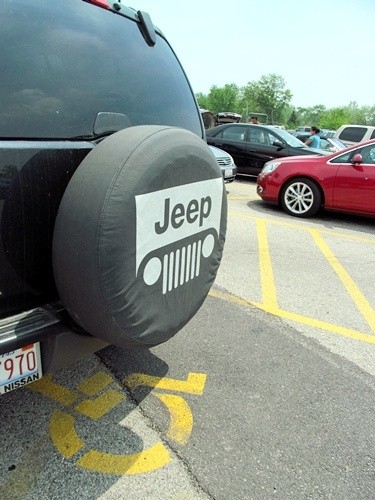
[{"left": 204, "top": 121, "right": 375, "bottom": 218}]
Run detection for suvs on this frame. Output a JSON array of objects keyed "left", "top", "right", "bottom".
[{"left": 0, "top": 0, "right": 227, "bottom": 392}]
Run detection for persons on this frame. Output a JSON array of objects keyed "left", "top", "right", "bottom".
[
  {"left": 249, "top": 117, "right": 262, "bottom": 143},
  {"left": 304, "top": 126, "right": 320, "bottom": 148}
]
